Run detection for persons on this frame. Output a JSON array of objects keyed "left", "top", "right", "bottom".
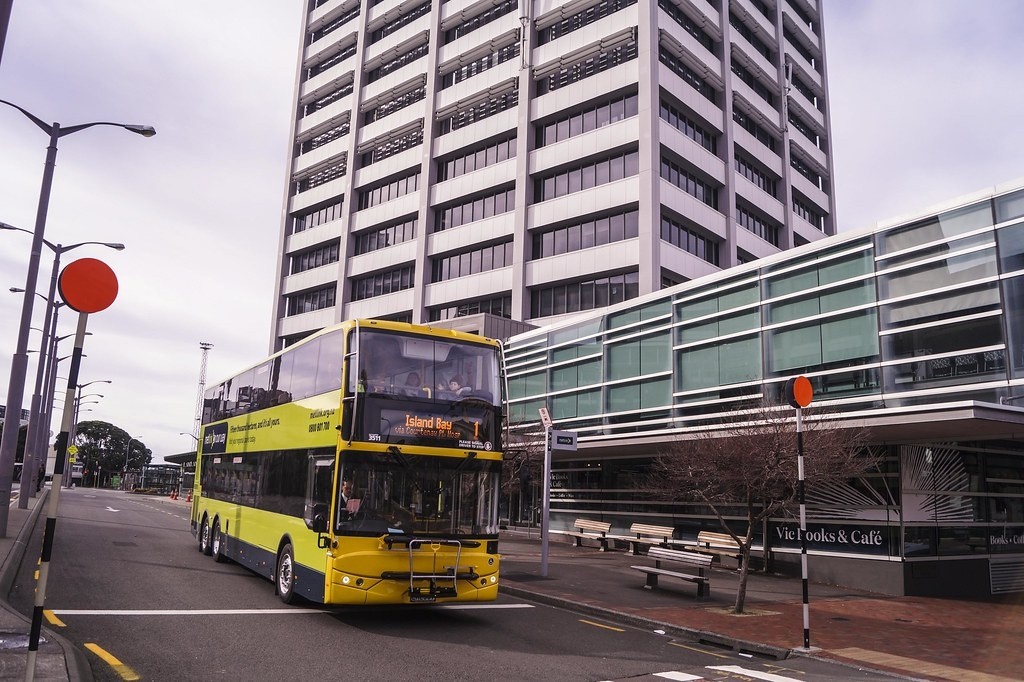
[
  {"left": 36, "top": 463, "right": 45, "bottom": 492},
  {"left": 437, "top": 375, "right": 472, "bottom": 401},
  {"left": 366, "top": 374, "right": 388, "bottom": 392},
  {"left": 401, "top": 372, "right": 427, "bottom": 397},
  {"left": 335, "top": 478, "right": 365, "bottom": 523}
]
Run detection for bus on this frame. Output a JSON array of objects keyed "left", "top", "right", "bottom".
[{"left": 189, "top": 318, "right": 531, "bottom": 605}]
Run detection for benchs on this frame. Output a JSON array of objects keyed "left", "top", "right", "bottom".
[{"left": 561, "top": 518, "right": 754, "bottom": 598}]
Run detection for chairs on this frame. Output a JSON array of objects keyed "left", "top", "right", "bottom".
[
  {"left": 383, "top": 386, "right": 408, "bottom": 397},
  {"left": 418, "top": 383, "right": 439, "bottom": 400},
  {"left": 212, "top": 397, "right": 278, "bottom": 421}
]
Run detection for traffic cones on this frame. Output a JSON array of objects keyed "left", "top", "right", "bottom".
[{"left": 170, "top": 489, "right": 191, "bottom": 502}]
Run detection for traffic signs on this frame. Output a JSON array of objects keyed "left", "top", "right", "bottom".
[{"left": 552, "top": 430, "right": 577, "bottom": 452}]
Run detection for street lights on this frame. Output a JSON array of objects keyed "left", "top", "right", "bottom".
[
  {"left": 123, "top": 436, "right": 142, "bottom": 490},
  {"left": 180, "top": 433, "right": 198, "bottom": 440},
  {"left": 0, "top": 99, "right": 157, "bottom": 537}
]
